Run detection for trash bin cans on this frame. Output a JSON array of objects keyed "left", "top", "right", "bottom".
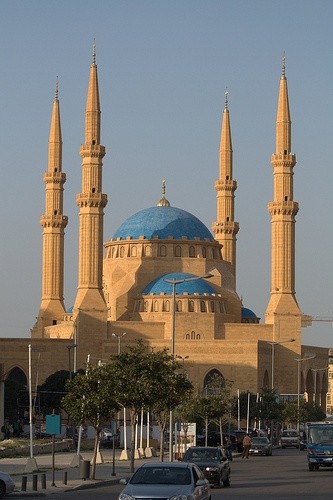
[{"left": 79, "top": 460, "right": 90, "bottom": 479}]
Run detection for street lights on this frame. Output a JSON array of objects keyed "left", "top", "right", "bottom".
[
  {"left": 163, "top": 273, "right": 215, "bottom": 462},
  {"left": 111, "top": 332, "right": 128, "bottom": 356},
  {"left": 66, "top": 343, "right": 79, "bottom": 380},
  {"left": 294, "top": 355, "right": 317, "bottom": 432},
  {"left": 311, "top": 367, "right": 330, "bottom": 407},
  {"left": 267, "top": 339, "right": 295, "bottom": 393}
]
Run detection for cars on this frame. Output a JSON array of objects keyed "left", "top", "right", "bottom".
[
  {"left": 82, "top": 425, "right": 116, "bottom": 448},
  {"left": 178, "top": 446, "right": 231, "bottom": 488},
  {"left": 118, "top": 461, "right": 215, "bottom": 500},
  {"left": 196, "top": 431, "right": 235, "bottom": 447},
  {"left": 35, "top": 428, "right": 53, "bottom": 439},
  {"left": 299, "top": 436, "right": 307, "bottom": 451},
  {"left": 0, "top": 471, "right": 15, "bottom": 498},
  {"left": 249, "top": 437, "right": 273, "bottom": 457}
]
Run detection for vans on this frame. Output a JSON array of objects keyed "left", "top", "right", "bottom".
[{"left": 280, "top": 430, "right": 300, "bottom": 449}]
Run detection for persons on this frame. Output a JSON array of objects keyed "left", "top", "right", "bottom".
[
  {"left": 23, "top": 410, "right": 30, "bottom": 425},
  {"left": 1, "top": 419, "right": 11, "bottom": 440},
  {"left": 320, "top": 431, "right": 328, "bottom": 440},
  {"left": 223, "top": 435, "right": 230, "bottom": 445},
  {"left": 241, "top": 434, "right": 251, "bottom": 458},
  {"left": 12, "top": 419, "right": 24, "bottom": 438},
  {"left": 250, "top": 428, "right": 257, "bottom": 437}
]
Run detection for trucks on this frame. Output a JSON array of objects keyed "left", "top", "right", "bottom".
[{"left": 305, "top": 421, "right": 333, "bottom": 472}]
